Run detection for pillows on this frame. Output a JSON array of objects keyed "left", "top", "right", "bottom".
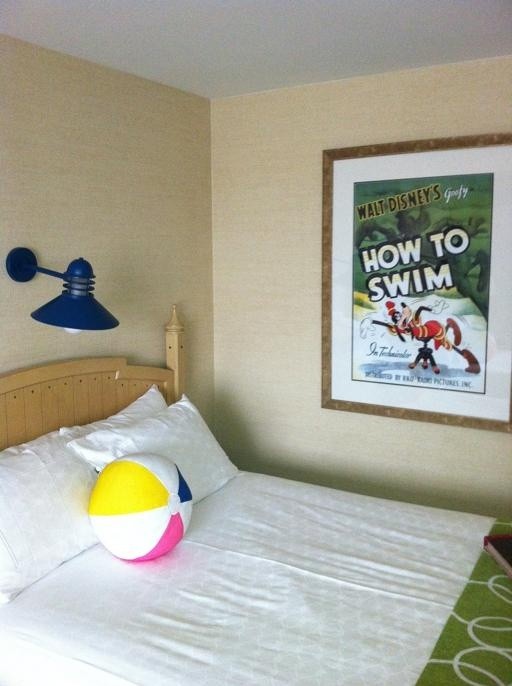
[
  {"left": 0, "top": 431, "right": 102, "bottom": 601},
  {"left": 57, "top": 385, "right": 167, "bottom": 440},
  {"left": 69, "top": 394, "right": 240, "bottom": 507}
]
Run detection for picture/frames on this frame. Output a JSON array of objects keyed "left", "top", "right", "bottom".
[{"left": 322, "top": 131, "right": 512, "bottom": 435}]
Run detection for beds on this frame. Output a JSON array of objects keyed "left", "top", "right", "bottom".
[{"left": 0, "top": 305, "right": 512, "bottom": 685}]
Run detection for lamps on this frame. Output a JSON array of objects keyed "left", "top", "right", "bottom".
[{"left": 4, "top": 246, "right": 119, "bottom": 334}]
